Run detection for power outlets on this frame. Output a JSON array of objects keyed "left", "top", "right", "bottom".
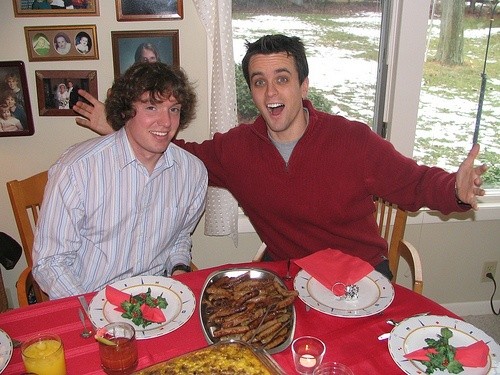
[{"left": 482, "top": 262, "right": 496, "bottom": 282}]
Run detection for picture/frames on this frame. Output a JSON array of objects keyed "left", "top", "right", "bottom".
[
  {"left": 12, "top": 0, "right": 100, "bottom": 17},
  {"left": 115, "top": 0, "right": 184, "bottom": 21},
  {"left": 111, "top": 29, "right": 180, "bottom": 83},
  {"left": 0, "top": 60, "right": 34, "bottom": 137},
  {"left": 24, "top": 25, "right": 99, "bottom": 62},
  {"left": 35, "top": 70, "right": 99, "bottom": 116}
]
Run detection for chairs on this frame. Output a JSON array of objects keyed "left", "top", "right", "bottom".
[
  {"left": 8, "top": 172, "right": 199, "bottom": 309},
  {"left": 251, "top": 197, "right": 423, "bottom": 295}
]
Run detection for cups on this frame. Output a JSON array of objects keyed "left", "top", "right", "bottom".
[
  {"left": 312, "top": 361, "right": 354, "bottom": 375},
  {"left": 291, "top": 335, "right": 326, "bottom": 375},
  {"left": 98, "top": 322, "right": 138, "bottom": 375},
  {"left": 21, "top": 332, "right": 67, "bottom": 375}
]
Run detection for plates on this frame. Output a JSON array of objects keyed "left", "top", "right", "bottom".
[
  {"left": 0, "top": 328, "right": 13, "bottom": 374},
  {"left": 387, "top": 315, "right": 500, "bottom": 375},
  {"left": 87, "top": 276, "right": 196, "bottom": 340},
  {"left": 293, "top": 268, "right": 397, "bottom": 316},
  {"left": 198, "top": 268, "right": 296, "bottom": 355}
]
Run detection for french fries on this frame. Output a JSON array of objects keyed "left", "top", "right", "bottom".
[{"left": 205, "top": 274, "right": 299, "bottom": 352}]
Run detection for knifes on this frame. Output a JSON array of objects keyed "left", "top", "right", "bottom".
[{"left": 378, "top": 311, "right": 430, "bottom": 341}]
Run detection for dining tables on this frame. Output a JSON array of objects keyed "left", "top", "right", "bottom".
[{"left": 0, "top": 258, "right": 463, "bottom": 375}]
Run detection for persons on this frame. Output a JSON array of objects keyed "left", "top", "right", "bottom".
[
  {"left": 56, "top": 35, "right": 71, "bottom": 54},
  {"left": 73, "top": 34, "right": 488, "bottom": 280},
  {"left": 135, "top": 42, "right": 157, "bottom": 63},
  {"left": 32, "top": 62, "right": 208, "bottom": 301},
  {"left": 38, "top": 38, "right": 45, "bottom": 47},
  {"left": 56, "top": 80, "right": 79, "bottom": 109},
  {"left": 0, "top": 73, "right": 27, "bottom": 132},
  {"left": 77, "top": 36, "right": 88, "bottom": 52}
]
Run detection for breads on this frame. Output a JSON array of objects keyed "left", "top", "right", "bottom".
[{"left": 133, "top": 342, "right": 270, "bottom": 375}]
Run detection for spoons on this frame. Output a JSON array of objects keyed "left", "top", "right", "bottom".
[
  {"left": 77, "top": 308, "right": 92, "bottom": 339},
  {"left": 283, "top": 259, "right": 293, "bottom": 279}
]
